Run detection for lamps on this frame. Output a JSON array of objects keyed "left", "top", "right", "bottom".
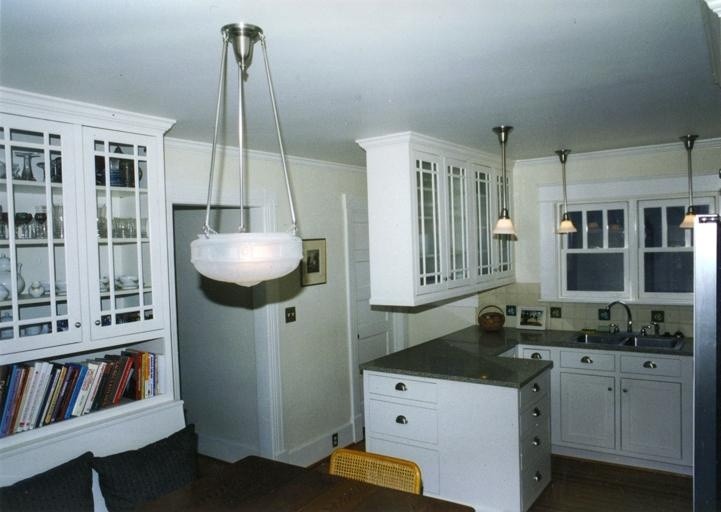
[
  {"left": 489, "top": 127, "right": 519, "bottom": 235},
  {"left": 191, "top": 22, "right": 303, "bottom": 288},
  {"left": 555, "top": 149, "right": 578, "bottom": 233},
  {"left": 678, "top": 134, "right": 701, "bottom": 228}
]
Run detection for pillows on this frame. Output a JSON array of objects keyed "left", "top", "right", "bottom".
[
  {"left": 93, "top": 422, "right": 201, "bottom": 512},
  {"left": 1, "top": 451, "right": 93, "bottom": 512}
]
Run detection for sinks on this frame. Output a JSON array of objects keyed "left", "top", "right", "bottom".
[
  {"left": 622, "top": 335, "right": 686, "bottom": 351},
  {"left": 574, "top": 330, "right": 628, "bottom": 345}
]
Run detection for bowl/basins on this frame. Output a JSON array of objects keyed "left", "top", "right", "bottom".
[
  {"left": 120, "top": 277, "right": 128, "bottom": 284},
  {"left": 41, "top": 280, "right": 51, "bottom": 295},
  {"left": 26, "top": 326, "right": 41, "bottom": 335},
  {"left": 55, "top": 281, "right": 66, "bottom": 295}
]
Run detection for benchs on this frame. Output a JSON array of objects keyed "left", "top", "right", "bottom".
[{"left": 2, "top": 400, "right": 188, "bottom": 512}]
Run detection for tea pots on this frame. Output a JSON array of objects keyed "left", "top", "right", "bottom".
[{"left": 0, "top": 252, "right": 25, "bottom": 300}]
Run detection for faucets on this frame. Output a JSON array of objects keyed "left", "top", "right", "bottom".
[
  {"left": 650, "top": 321, "right": 660, "bottom": 337},
  {"left": 607, "top": 299, "right": 634, "bottom": 333}
]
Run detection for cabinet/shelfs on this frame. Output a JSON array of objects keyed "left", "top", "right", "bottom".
[
  {"left": 555, "top": 343, "right": 694, "bottom": 476},
  {"left": 355, "top": 130, "right": 517, "bottom": 307},
  {"left": 0, "top": 87, "right": 180, "bottom": 449},
  {"left": 362, "top": 370, "right": 440, "bottom": 497},
  {"left": 439, "top": 368, "right": 551, "bottom": 512},
  {"left": 517, "top": 343, "right": 555, "bottom": 366}
]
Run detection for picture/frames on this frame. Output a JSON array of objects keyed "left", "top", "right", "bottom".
[{"left": 300, "top": 238, "right": 328, "bottom": 287}]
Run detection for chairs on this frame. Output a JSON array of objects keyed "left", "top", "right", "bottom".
[{"left": 327, "top": 448, "right": 423, "bottom": 494}]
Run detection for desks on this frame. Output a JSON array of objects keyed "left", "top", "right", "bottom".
[{"left": 115, "top": 454, "right": 476, "bottom": 511}]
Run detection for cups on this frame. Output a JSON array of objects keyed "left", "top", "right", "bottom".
[{"left": 97, "top": 217, "right": 148, "bottom": 239}]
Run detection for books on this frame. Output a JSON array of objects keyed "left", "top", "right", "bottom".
[{"left": 0, "top": 349, "right": 166, "bottom": 438}]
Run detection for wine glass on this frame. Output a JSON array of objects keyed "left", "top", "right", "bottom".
[{"left": 0, "top": 212, "right": 47, "bottom": 239}]
